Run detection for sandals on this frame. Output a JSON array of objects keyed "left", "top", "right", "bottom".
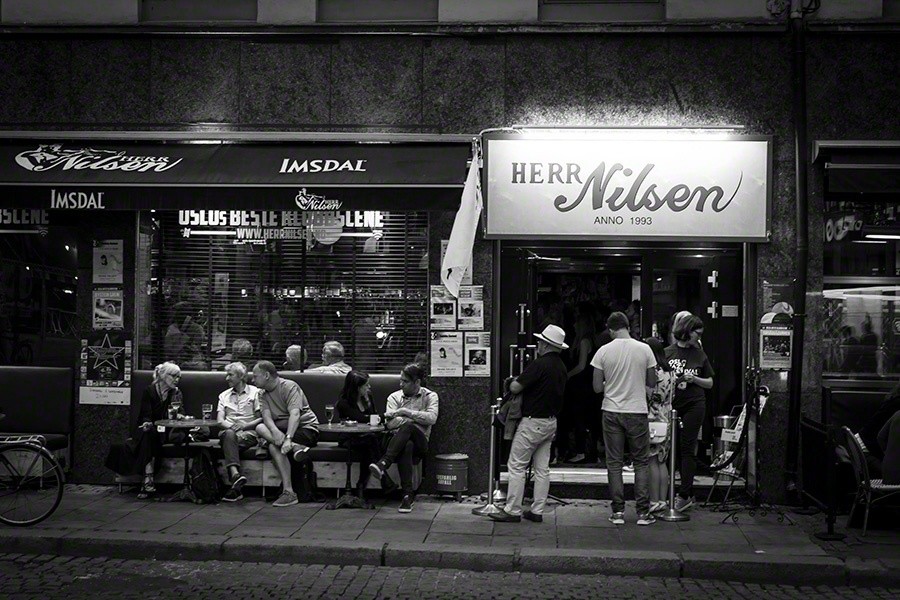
[
  {"left": 137, "top": 485, "right": 149, "bottom": 499},
  {"left": 649, "top": 501, "right": 667, "bottom": 512},
  {"left": 143, "top": 472, "right": 156, "bottom": 493}
]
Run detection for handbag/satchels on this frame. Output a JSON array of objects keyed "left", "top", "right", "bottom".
[{"left": 648, "top": 422, "right": 668, "bottom": 444}]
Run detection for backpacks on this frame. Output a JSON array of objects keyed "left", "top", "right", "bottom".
[{"left": 189, "top": 452, "right": 223, "bottom": 503}]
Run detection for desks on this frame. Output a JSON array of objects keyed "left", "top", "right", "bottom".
[
  {"left": 316, "top": 422, "right": 385, "bottom": 508},
  {"left": 154, "top": 419, "right": 221, "bottom": 503}
]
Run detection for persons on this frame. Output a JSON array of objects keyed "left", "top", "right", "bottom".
[
  {"left": 136, "top": 362, "right": 187, "bottom": 498},
  {"left": 838, "top": 321, "right": 879, "bottom": 374},
  {"left": 337, "top": 370, "right": 398, "bottom": 500},
  {"left": 252, "top": 360, "right": 321, "bottom": 506},
  {"left": 217, "top": 362, "right": 263, "bottom": 501},
  {"left": 369, "top": 364, "right": 439, "bottom": 512},
  {"left": 173, "top": 301, "right": 353, "bottom": 375},
  {"left": 487, "top": 300, "right": 715, "bottom": 524}
]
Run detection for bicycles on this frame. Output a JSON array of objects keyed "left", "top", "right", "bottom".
[{"left": 0, "top": 413, "right": 66, "bottom": 528}]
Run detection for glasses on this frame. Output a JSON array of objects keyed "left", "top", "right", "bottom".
[
  {"left": 694, "top": 330, "right": 703, "bottom": 337},
  {"left": 167, "top": 372, "right": 182, "bottom": 380}
]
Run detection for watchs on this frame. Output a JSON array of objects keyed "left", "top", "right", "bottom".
[{"left": 284, "top": 434, "right": 293, "bottom": 440}]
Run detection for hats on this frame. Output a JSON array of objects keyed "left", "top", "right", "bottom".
[{"left": 534, "top": 325, "right": 570, "bottom": 349}]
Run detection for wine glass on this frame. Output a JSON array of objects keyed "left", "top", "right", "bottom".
[
  {"left": 171, "top": 402, "right": 181, "bottom": 422},
  {"left": 325, "top": 404, "right": 335, "bottom": 427}
]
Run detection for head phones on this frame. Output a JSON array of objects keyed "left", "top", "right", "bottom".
[{"left": 678, "top": 315, "right": 696, "bottom": 342}]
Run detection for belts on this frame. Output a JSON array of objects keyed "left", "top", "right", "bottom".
[{"left": 523, "top": 414, "right": 555, "bottom": 419}]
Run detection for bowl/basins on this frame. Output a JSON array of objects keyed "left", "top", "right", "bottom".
[{"left": 346, "top": 421, "right": 357, "bottom": 427}]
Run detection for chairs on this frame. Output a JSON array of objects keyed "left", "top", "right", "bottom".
[{"left": 843, "top": 425, "right": 900, "bottom": 537}]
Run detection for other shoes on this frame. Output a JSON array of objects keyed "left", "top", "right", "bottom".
[
  {"left": 222, "top": 491, "right": 243, "bottom": 503},
  {"left": 380, "top": 479, "right": 399, "bottom": 493},
  {"left": 622, "top": 462, "right": 635, "bottom": 472},
  {"left": 356, "top": 482, "right": 367, "bottom": 499},
  {"left": 231, "top": 473, "right": 246, "bottom": 491},
  {"left": 488, "top": 509, "right": 521, "bottom": 522},
  {"left": 570, "top": 453, "right": 585, "bottom": 461},
  {"left": 522, "top": 511, "right": 543, "bottom": 522}
]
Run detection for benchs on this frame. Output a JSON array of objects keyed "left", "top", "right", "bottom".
[
  {"left": 130, "top": 369, "right": 424, "bottom": 497},
  {"left": 0, "top": 365, "right": 75, "bottom": 488}
]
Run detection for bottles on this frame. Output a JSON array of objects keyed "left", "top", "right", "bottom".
[{"left": 168, "top": 405, "right": 174, "bottom": 420}]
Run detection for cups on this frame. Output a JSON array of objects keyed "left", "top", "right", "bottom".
[
  {"left": 370, "top": 415, "right": 381, "bottom": 426},
  {"left": 202, "top": 404, "right": 212, "bottom": 422}
]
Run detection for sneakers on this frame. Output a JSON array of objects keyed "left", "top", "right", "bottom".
[
  {"left": 690, "top": 497, "right": 696, "bottom": 506},
  {"left": 609, "top": 513, "right": 624, "bottom": 524},
  {"left": 674, "top": 494, "right": 692, "bottom": 512},
  {"left": 273, "top": 490, "right": 299, "bottom": 507},
  {"left": 291, "top": 444, "right": 310, "bottom": 462},
  {"left": 637, "top": 513, "right": 656, "bottom": 525},
  {"left": 398, "top": 496, "right": 415, "bottom": 512},
  {"left": 370, "top": 459, "right": 390, "bottom": 479}
]
